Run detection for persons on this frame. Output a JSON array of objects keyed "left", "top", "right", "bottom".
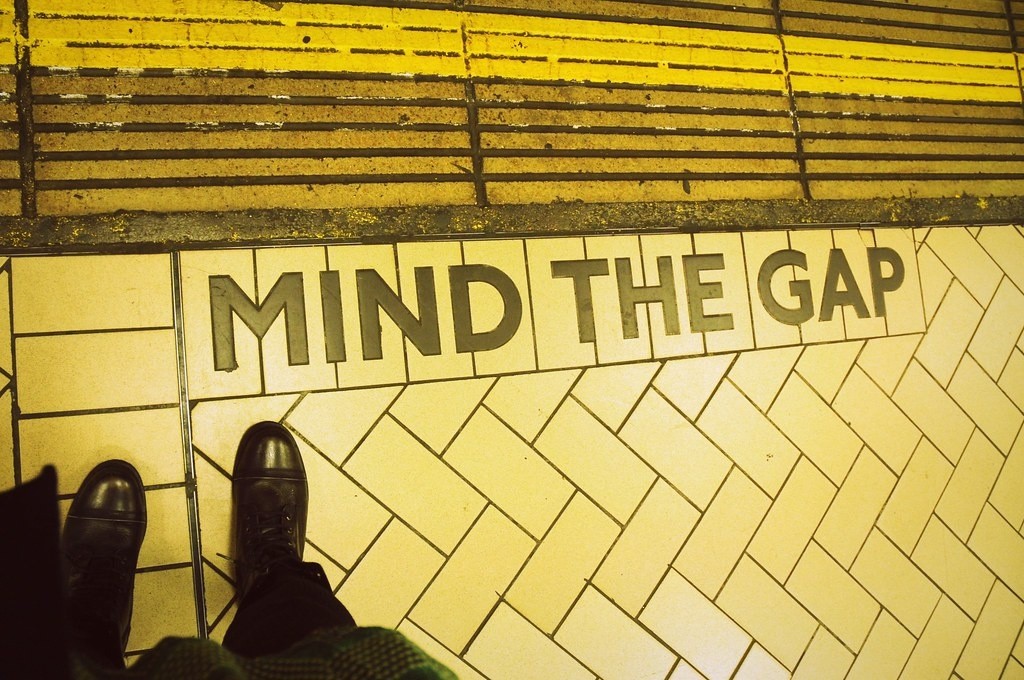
[{"left": 0, "top": 421, "right": 460, "bottom": 680}]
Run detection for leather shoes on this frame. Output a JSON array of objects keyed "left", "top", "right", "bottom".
[
  {"left": 231, "top": 421, "right": 309, "bottom": 608},
  {"left": 61, "top": 459, "right": 146, "bottom": 655}
]
